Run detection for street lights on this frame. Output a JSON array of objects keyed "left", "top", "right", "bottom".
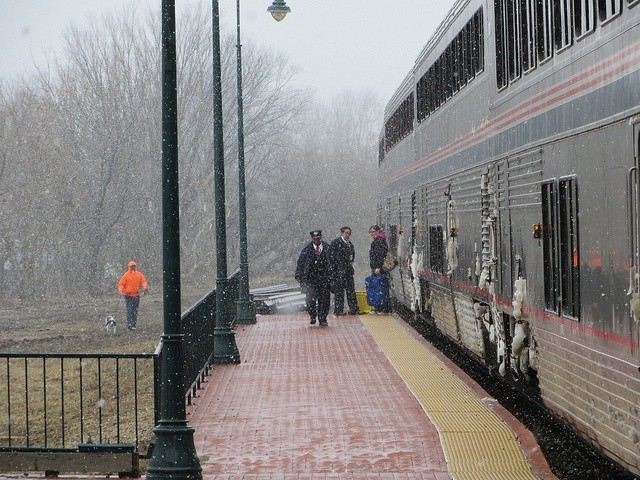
[{"left": 235, "top": 0, "right": 292, "bottom": 325}]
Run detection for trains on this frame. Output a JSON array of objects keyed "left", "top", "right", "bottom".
[{"left": 377, "top": 0, "right": 640, "bottom": 477}]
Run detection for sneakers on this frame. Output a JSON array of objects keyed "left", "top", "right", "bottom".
[{"left": 127, "top": 322, "right": 135, "bottom": 330}]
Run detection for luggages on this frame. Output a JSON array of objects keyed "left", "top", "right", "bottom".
[{"left": 365, "top": 269, "right": 389, "bottom": 306}]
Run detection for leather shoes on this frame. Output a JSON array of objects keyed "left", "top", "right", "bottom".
[
  {"left": 333, "top": 310, "right": 348, "bottom": 316},
  {"left": 319, "top": 319, "right": 327, "bottom": 327},
  {"left": 348, "top": 308, "right": 367, "bottom": 316},
  {"left": 310, "top": 315, "right": 316, "bottom": 324}
]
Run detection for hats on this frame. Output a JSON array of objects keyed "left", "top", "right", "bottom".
[{"left": 309, "top": 230, "right": 322, "bottom": 239}]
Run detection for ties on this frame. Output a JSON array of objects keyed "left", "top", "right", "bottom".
[{"left": 315, "top": 246, "right": 321, "bottom": 259}]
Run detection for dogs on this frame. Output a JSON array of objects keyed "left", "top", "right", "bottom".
[{"left": 106, "top": 316, "right": 117, "bottom": 334}]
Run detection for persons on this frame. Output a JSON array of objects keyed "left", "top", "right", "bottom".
[
  {"left": 367, "top": 225, "right": 391, "bottom": 315},
  {"left": 328, "top": 226, "right": 365, "bottom": 315},
  {"left": 295, "top": 230, "right": 332, "bottom": 327},
  {"left": 118, "top": 261, "right": 148, "bottom": 332}
]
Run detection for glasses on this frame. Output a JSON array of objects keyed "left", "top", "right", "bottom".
[{"left": 368, "top": 230, "right": 377, "bottom": 234}]
